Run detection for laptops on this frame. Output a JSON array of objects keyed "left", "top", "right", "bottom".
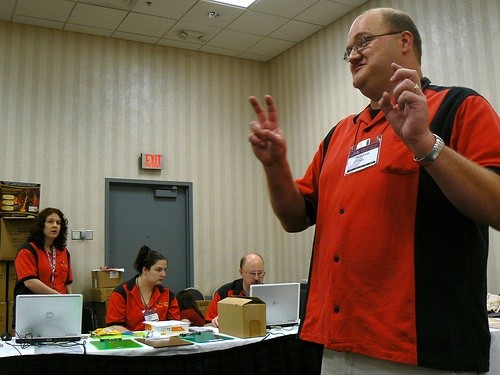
[
  {"left": 14, "top": 293, "right": 83, "bottom": 343},
  {"left": 248, "top": 282, "right": 301, "bottom": 326}
]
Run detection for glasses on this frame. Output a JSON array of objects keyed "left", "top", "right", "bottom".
[
  {"left": 242, "top": 268, "right": 265, "bottom": 278},
  {"left": 343, "top": 32, "right": 404, "bottom": 63}
]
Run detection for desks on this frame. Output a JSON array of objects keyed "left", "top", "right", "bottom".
[{"left": 0, "top": 324, "right": 324, "bottom": 375}]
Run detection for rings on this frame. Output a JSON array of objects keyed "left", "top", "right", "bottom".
[{"left": 411, "top": 83, "right": 420, "bottom": 92}]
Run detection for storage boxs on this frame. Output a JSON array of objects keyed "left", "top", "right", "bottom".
[
  {"left": 90, "top": 268, "right": 122, "bottom": 327},
  {"left": 0, "top": 181, "right": 41, "bottom": 340},
  {"left": 217, "top": 294, "right": 267, "bottom": 339},
  {"left": 143, "top": 320, "right": 189, "bottom": 334}
]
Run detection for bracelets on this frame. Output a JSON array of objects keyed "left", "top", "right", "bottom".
[{"left": 412, "top": 133, "right": 445, "bottom": 166}]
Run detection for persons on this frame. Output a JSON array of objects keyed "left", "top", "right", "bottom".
[
  {"left": 247, "top": 6, "right": 500, "bottom": 375},
  {"left": 204, "top": 253, "right": 266, "bottom": 328},
  {"left": 175, "top": 290, "right": 206, "bottom": 327},
  {"left": 105, "top": 244, "right": 181, "bottom": 331},
  {"left": 12, "top": 208, "right": 74, "bottom": 330}
]
